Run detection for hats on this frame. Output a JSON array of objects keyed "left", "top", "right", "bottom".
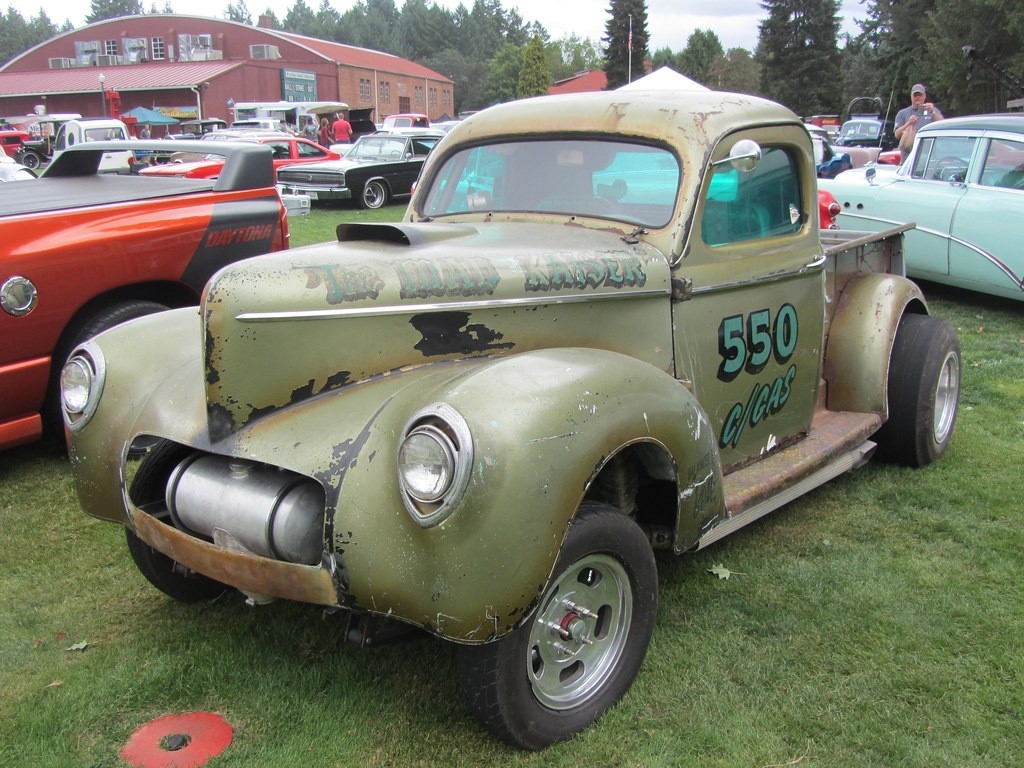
[{"left": 911, "top": 84, "right": 925, "bottom": 94}]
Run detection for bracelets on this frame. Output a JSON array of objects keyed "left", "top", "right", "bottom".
[{"left": 929, "top": 110, "right": 936, "bottom": 116}]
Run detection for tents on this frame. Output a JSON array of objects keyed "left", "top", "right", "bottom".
[{"left": 120, "top": 106, "right": 179, "bottom": 135}]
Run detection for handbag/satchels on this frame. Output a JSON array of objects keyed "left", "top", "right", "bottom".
[{"left": 899, "top": 125, "right": 917, "bottom": 149}]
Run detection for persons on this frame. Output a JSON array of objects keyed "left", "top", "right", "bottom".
[
  {"left": 301, "top": 116, "right": 319, "bottom": 145},
  {"left": 317, "top": 117, "right": 335, "bottom": 150},
  {"left": 892, "top": 84, "right": 944, "bottom": 166},
  {"left": 140, "top": 124, "right": 150, "bottom": 140},
  {"left": 279, "top": 119, "right": 299, "bottom": 137},
  {"left": 332, "top": 113, "right": 353, "bottom": 144}
]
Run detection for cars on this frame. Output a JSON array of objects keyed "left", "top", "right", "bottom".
[
  {"left": 57, "top": 87, "right": 964, "bottom": 750},
  {"left": 1, "top": 140, "right": 290, "bottom": 454},
  {"left": 836, "top": 119, "right": 896, "bottom": 151},
  {"left": 275, "top": 134, "right": 445, "bottom": 209},
  {"left": 1, "top": 101, "right": 462, "bottom": 175},
  {"left": 816, "top": 111, "right": 1023, "bottom": 302},
  {"left": 139, "top": 135, "right": 342, "bottom": 180}
]
